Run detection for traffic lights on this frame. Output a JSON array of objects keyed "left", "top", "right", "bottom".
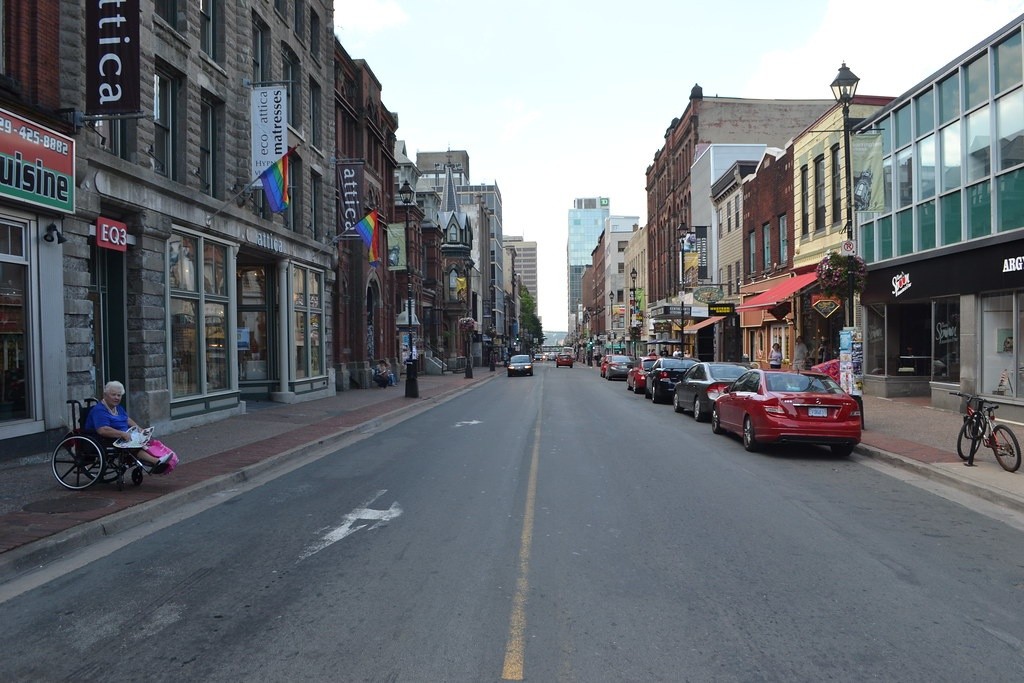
[
  {"left": 630, "top": 326, "right": 639, "bottom": 345},
  {"left": 516, "top": 333, "right": 519, "bottom": 341},
  {"left": 590, "top": 337, "right": 593, "bottom": 345}
]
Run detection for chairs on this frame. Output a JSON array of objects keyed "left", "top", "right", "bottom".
[{"left": 773, "top": 383, "right": 787, "bottom": 391}]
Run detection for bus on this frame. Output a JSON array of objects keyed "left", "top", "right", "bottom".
[{"left": 561, "top": 346, "right": 575, "bottom": 362}]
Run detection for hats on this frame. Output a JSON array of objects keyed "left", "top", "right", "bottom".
[{"left": 378, "top": 358, "right": 392, "bottom": 367}]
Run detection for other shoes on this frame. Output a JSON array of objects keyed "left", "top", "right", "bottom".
[
  {"left": 155, "top": 450, "right": 171, "bottom": 468},
  {"left": 392, "top": 383, "right": 398, "bottom": 387}
]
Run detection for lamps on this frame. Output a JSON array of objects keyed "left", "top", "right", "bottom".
[
  {"left": 279, "top": 212, "right": 289, "bottom": 227},
  {"left": 88, "top": 121, "right": 106, "bottom": 145},
  {"left": 232, "top": 184, "right": 246, "bottom": 205},
  {"left": 196, "top": 167, "right": 210, "bottom": 190},
  {"left": 249, "top": 192, "right": 265, "bottom": 213},
  {"left": 149, "top": 145, "right": 165, "bottom": 171},
  {"left": 44, "top": 223, "right": 68, "bottom": 245}
]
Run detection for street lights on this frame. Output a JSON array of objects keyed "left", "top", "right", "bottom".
[
  {"left": 462, "top": 254, "right": 473, "bottom": 378},
  {"left": 609, "top": 291, "right": 615, "bottom": 353},
  {"left": 397, "top": 179, "right": 419, "bottom": 397},
  {"left": 488, "top": 282, "right": 496, "bottom": 371},
  {"left": 830, "top": 62, "right": 865, "bottom": 430},
  {"left": 675, "top": 222, "right": 689, "bottom": 358},
  {"left": 630, "top": 268, "right": 638, "bottom": 358}
]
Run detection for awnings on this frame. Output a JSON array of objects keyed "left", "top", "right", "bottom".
[
  {"left": 735, "top": 272, "right": 819, "bottom": 312},
  {"left": 684, "top": 316, "right": 726, "bottom": 334}
]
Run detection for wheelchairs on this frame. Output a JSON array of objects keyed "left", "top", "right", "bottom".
[{"left": 51, "top": 397, "right": 170, "bottom": 491}]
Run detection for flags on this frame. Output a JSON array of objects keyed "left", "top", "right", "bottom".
[
  {"left": 259, "top": 154, "right": 289, "bottom": 213},
  {"left": 355, "top": 208, "right": 379, "bottom": 267}
]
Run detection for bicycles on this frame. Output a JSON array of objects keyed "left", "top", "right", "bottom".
[{"left": 948, "top": 391, "right": 1021, "bottom": 473}]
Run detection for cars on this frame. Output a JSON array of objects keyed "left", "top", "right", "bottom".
[
  {"left": 599, "top": 353, "right": 637, "bottom": 381},
  {"left": 555, "top": 353, "right": 575, "bottom": 368},
  {"left": 626, "top": 356, "right": 664, "bottom": 394},
  {"left": 506, "top": 354, "right": 535, "bottom": 377},
  {"left": 534, "top": 351, "right": 561, "bottom": 362},
  {"left": 644, "top": 356, "right": 702, "bottom": 404},
  {"left": 712, "top": 368, "right": 862, "bottom": 459},
  {"left": 673, "top": 362, "right": 759, "bottom": 422}
]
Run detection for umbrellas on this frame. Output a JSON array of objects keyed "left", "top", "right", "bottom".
[{"left": 643, "top": 338, "right": 692, "bottom": 345}]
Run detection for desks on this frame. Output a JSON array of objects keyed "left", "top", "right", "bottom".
[{"left": 900, "top": 355, "right": 931, "bottom": 376}]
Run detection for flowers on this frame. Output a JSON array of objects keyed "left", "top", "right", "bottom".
[
  {"left": 816, "top": 254, "right": 869, "bottom": 301},
  {"left": 458, "top": 317, "right": 474, "bottom": 333}
]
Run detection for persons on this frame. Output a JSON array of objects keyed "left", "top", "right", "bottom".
[
  {"left": 627, "top": 351, "right": 631, "bottom": 356},
  {"left": 373, "top": 360, "right": 398, "bottom": 389},
  {"left": 684, "top": 350, "right": 690, "bottom": 358},
  {"left": 673, "top": 347, "right": 682, "bottom": 356},
  {"left": 792, "top": 336, "right": 808, "bottom": 373},
  {"left": 85, "top": 381, "right": 174, "bottom": 467},
  {"left": 660, "top": 346, "right": 668, "bottom": 357},
  {"left": 650, "top": 349, "right": 656, "bottom": 356},
  {"left": 768, "top": 343, "right": 782, "bottom": 369}
]
